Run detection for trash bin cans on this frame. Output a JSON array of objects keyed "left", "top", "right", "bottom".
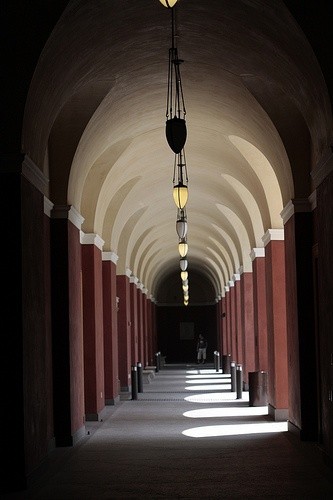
[
  {"left": 222, "top": 354, "right": 231, "bottom": 374},
  {"left": 248, "top": 370, "right": 270, "bottom": 407}
]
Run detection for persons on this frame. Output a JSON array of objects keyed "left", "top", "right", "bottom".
[{"left": 196, "top": 334, "right": 208, "bottom": 364}]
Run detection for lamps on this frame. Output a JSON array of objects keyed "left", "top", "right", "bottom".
[
  {"left": 180, "top": 255, "right": 189, "bottom": 306},
  {"left": 175, "top": 206, "right": 188, "bottom": 239},
  {"left": 172, "top": 146, "right": 190, "bottom": 213},
  {"left": 177, "top": 237, "right": 189, "bottom": 258},
  {"left": 164, "top": 5, "right": 189, "bottom": 156}
]
